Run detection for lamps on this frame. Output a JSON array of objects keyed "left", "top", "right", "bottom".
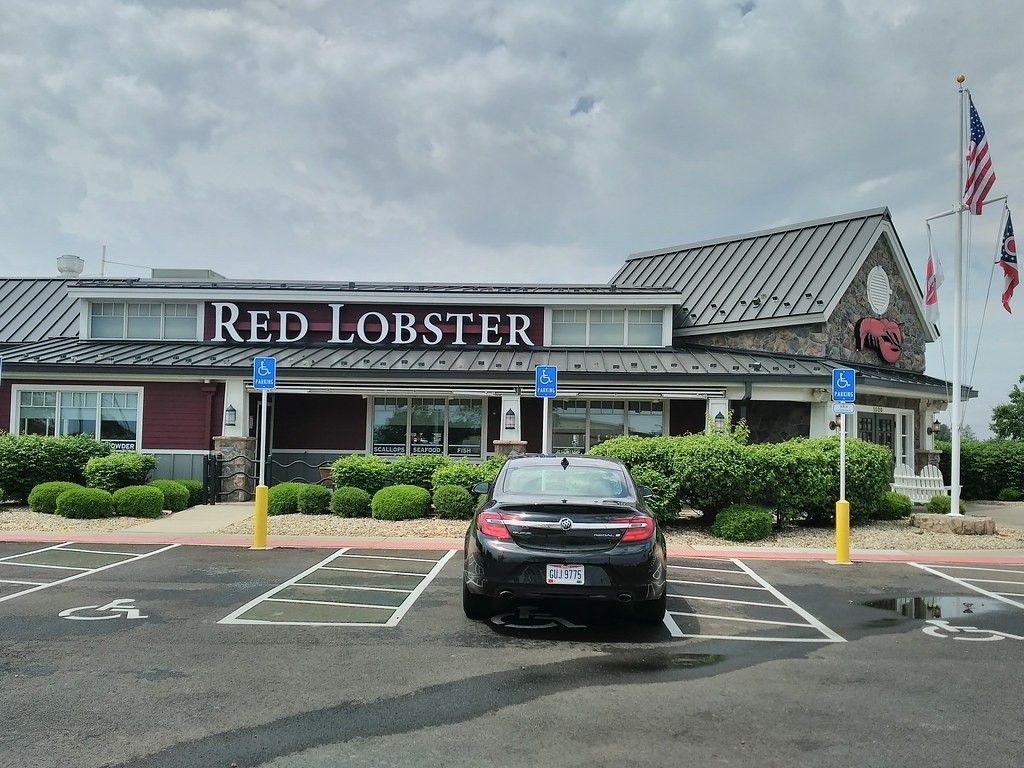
[
  {"left": 829, "top": 414, "right": 841, "bottom": 430},
  {"left": 224, "top": 404, "right": 236, "bottom": 426},
  {"left": 715, "top": 411, "right": 725, "bottom": 427},
  {"left": 505, "top": 409, "right": 516, "bottom": 429},
  {"left": 927, "top": 419, "right": 941, "bottom": 435}
]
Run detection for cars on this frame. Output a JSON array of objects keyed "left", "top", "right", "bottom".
[{"left": 460, "top": 452, "right": 667, "bottom": 625}]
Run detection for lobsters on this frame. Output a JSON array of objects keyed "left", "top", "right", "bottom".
[{"left": 859, "top": 317, "right": 902, "bottom": 363}]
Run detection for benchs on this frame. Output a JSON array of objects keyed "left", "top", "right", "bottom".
[{"left": 889, "top": 463, "right": 963, "bottom": 505}]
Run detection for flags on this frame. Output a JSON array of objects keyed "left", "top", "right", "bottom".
[
  {"left": 1000, "top": 210, "right": 1019, "bottom": 314},
  {"left": 963, "top": 94, "right": 996, "bottom": 215},
  {"left": 922, "top": 224, "right": 946, "bottom": 325}
]
